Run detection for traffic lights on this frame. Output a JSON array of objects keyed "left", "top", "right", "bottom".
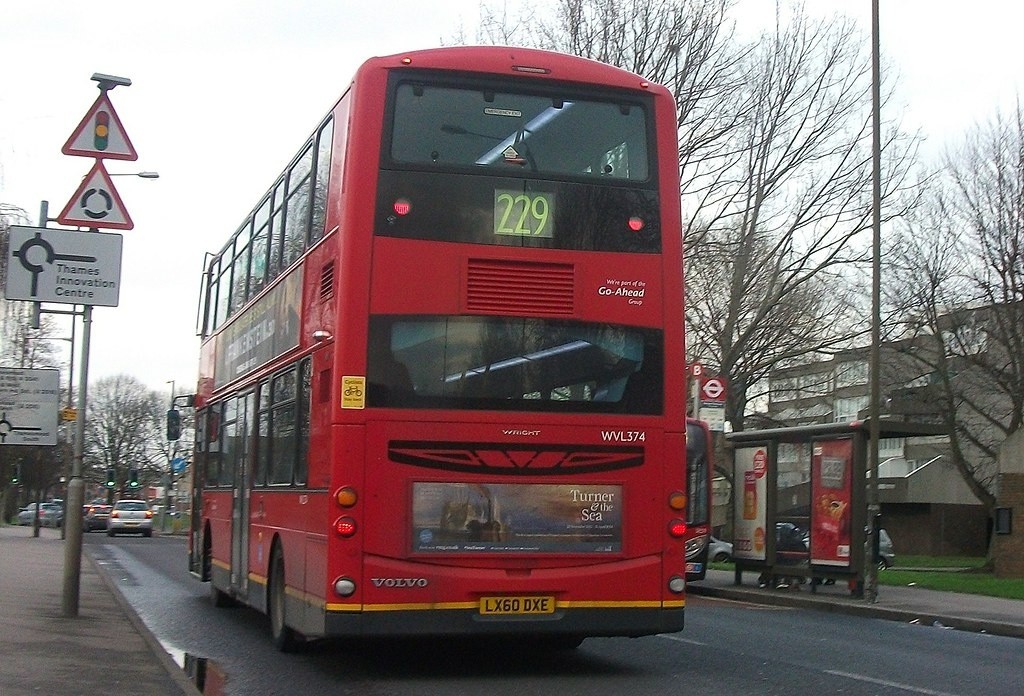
[
  {"left": 129, "top": 469, "right": 140, "bottom": 489},
  {"left": 60, "top": 475, "right": 67, "bottom": 485},
  {"left": 11, "top": 464, "right": 21, "bottom": 485},
  {"left": 106, "top": 469, "right": 116, "bottom": 489}
]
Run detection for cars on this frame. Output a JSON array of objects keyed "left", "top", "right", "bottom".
[
  {"left": 18, "top": 503, "right": 63, "bottom": 527},
  {"left": 106, "top": 498, "right": 154, "bottom": 538},
  {"left": 707, "top": 534, "right": 733, "bottom": 564},
  {"left": 48, "top": 497, "right": 115, "bottom": 533}
]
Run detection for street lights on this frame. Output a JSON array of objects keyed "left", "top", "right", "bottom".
[
  {"left": 21, "top": 172, "right": 161, "bottom": 539},
  {"left": 166, "top": 379, "right": 176, "bottom": 511}
]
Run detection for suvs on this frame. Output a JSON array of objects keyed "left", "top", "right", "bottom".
[{"left": 774, "top": 522, "right": 896, "bottom": 572}]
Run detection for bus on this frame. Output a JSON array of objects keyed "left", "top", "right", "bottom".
[
  {"left": 163, "top": 45, "right": 691, "bottom": 653},
  {"left": 686, "top": 418, "right": 716, "bottom": 582}
]
